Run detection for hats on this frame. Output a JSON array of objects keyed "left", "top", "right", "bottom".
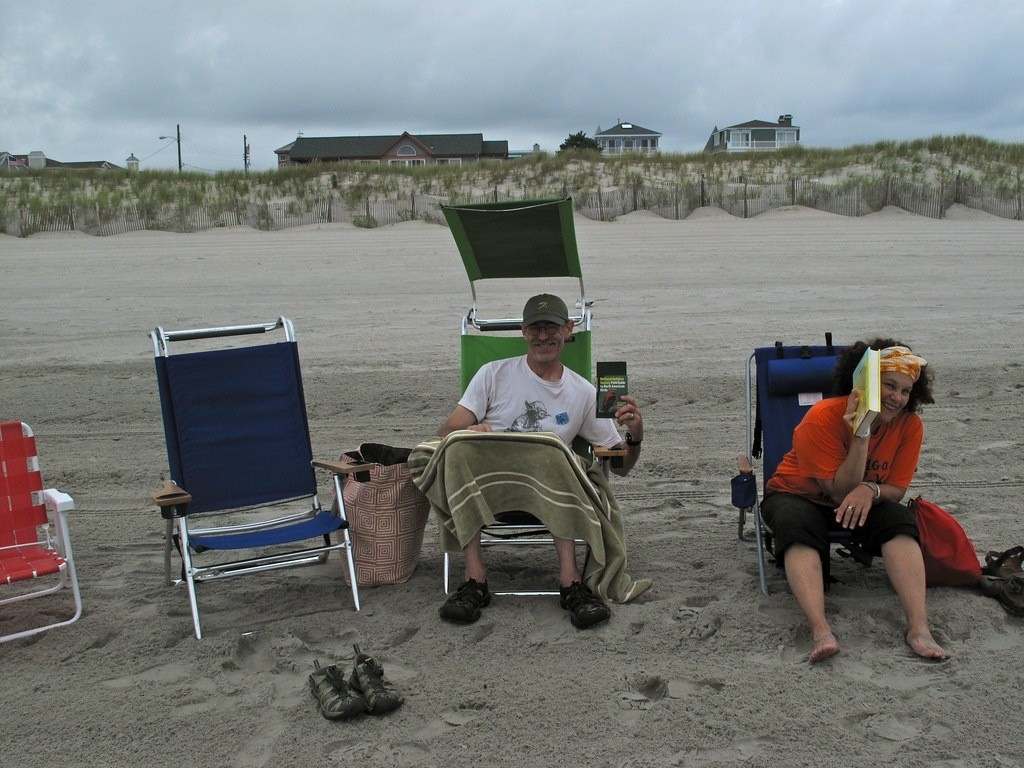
[{"left": 523, "top": 293, "right": 570, "bottom": 327}]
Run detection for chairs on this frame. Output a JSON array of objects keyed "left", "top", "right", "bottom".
[
  {"left": 149, "top": 316, "right": 376, "bottom": 640},
  {"left": 0, "top": 421, "right": 81, "bottom": 645},
  {"left": 439, "top": 195, "right": 629, "bottom": 598},
  {"left": 731, "top": 332, "right": 855, "bottom": 596}
]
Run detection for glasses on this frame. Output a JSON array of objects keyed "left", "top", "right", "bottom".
[{"left": 523, "top": 322, "right": 561, "bottom": 336}]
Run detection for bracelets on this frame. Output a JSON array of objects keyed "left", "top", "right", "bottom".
[{"left": 859, "top": 481, "right": 881, "bottom": 500}]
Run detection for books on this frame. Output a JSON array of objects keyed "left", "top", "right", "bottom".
[
  {"left": 595, "top": 360, "right": 629, "bottom": 419},
  {"left": 853, "top": 346, "right": 882, "bottom": 440}
]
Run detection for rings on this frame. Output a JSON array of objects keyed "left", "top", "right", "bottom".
[
  {"left": 846, "top": 407, "right": 849, "bottom": 413},
  {"left": 848, "top": 506, "right": 855, "bottom": 511},
  {"left": 631, "top": 413, "right": 634, "bottom": 419}
]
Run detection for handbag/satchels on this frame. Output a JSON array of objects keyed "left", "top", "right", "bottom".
[
  {"left": 907, "top": 494, "right": 981, "bottom": 588},
  {"left": 979, "top": 545, "right": 1024, "bottom": 619}
]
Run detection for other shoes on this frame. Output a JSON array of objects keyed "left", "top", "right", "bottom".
[
  {"left": 438, "top": 576, "right": 491, "bottom": 625},
  {"left": 559, "top": 579, "right": 610, "bottom": 629}
]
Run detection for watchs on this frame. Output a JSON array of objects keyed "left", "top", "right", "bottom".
[{"left": 625, "top": 432, "right": 644, "bottom": 447}]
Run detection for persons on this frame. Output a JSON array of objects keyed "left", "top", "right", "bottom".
[
  {"left": 438, "top": 290, "right": 645, "bottom": 630},
  {"left": 758, "top": 337, "right": 949, "bottom": 662}
]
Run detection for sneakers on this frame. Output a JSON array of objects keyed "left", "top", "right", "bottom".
[
  {"left": 349, "top": 643, "right": 405, "bottom": 716},
  {"left": 309, "top": 659, "right": 364, "bottom": 721}
]
